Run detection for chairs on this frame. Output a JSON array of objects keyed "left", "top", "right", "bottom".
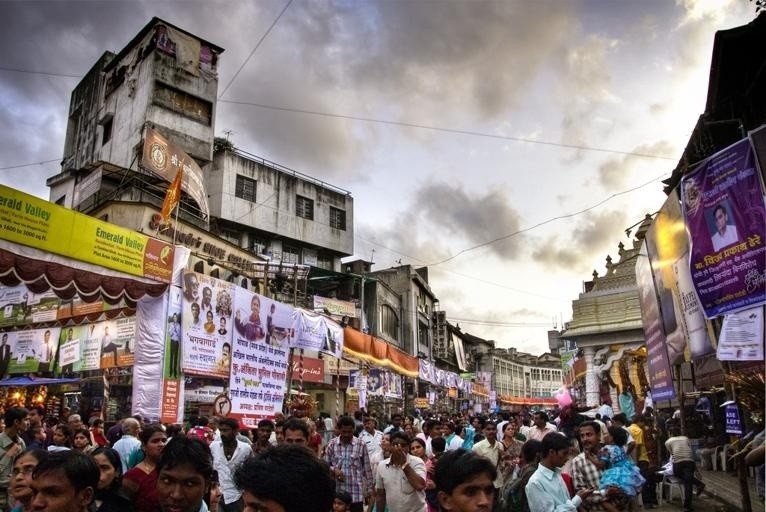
[{"left": 660, "top": 474, "right": 684, "bottom": 506}]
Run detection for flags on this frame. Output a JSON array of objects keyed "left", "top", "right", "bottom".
[{"left": 160, "top": 160, "right": 183, "bottom": 227}]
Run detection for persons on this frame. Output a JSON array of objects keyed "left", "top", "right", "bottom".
[
  {"left": 101, "top": 326, "right": 122, "bottom": 366},
  {"left": 167, "top": 312, "right": 181, "bottom": 379},
  {"left": 184, "top": 273, "right": 200, "bottom": 303},
  {"left": 0, "top": 333, "right": 11, "bottom": 381},
  {"left": 217, "top": 342, "right": 231, "bottom": 369},
  {"left": 1, "top": 386, "right": 765, "bottom": 510},
  {"left": 217, "top": 317, "right": 228, "bottom": 336},
  {"left": 188, "top": 302, "right": 203, "bottom": 329},
  {"left": 265, "top": 303, "right": 276, "bottom": 344},
  {"left": 37, "top": 330, "right": 53, "bottom": 372},
  {"left": 201, "top": 287, "right": 213, "bottom": 311},
  {"left": 204, "top": 311, "right": 216, "bottom": 333},
  {"left": 710, "top": 204, "right": 739, "bottom": 251},
  {"left": 61, "top": 328, "right": 74, "bottom": 373},
  {"left": 235, "top": 295, "right": 265, "bottom": 342}
]
